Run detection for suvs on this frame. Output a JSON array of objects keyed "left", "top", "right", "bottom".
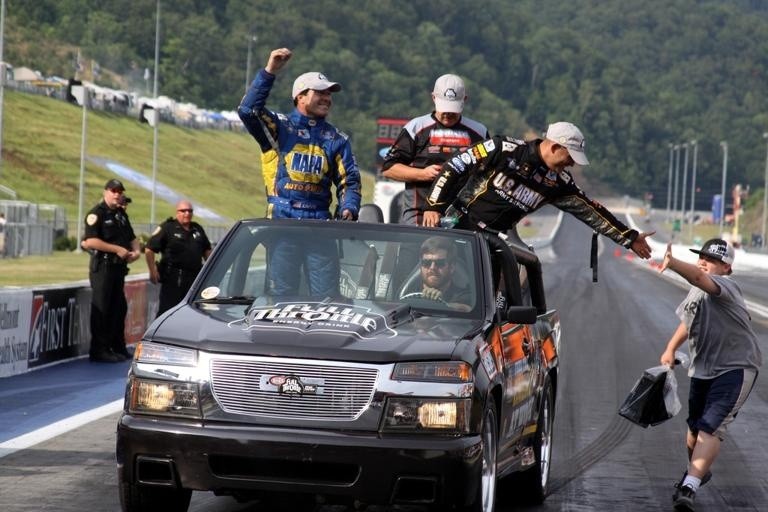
[{"left": 116, "top": 204, "right": 560, "bottom": 509}]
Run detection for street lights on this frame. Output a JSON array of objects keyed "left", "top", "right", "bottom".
[
  {"left": 679, "top": 142, "right": 689, "bottom": 237},
  {"left": 670, "top": 144, "right": 679, "bottom": 239},
  {"left": 719, "top": 140, "right": 729, "bottom": 239},
  {"left": 142, "top": 108, "right": 158, "bottom": 229},
  {"left": 761, "top": 132, "right": 768, "bottom": 251},
  {"left": 688, "top": 139, "right": 697, "bottom": 239},
  {"left": 242, "top": 34, "right": 258, "bottom": 94},
  {"left": 666, "top": 142, "right": 673, "bottom": 235},
  {"left": 71, "top": 85, "right": 86, "bottom": 255}
]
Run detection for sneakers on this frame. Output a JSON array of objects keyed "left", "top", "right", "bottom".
[{"left": 673, "top": 469, "right": 712, "bottom": 511}]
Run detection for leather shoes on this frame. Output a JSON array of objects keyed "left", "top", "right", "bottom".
[{"left": 96, "top": 351, "right": 133, "bottom": 363}]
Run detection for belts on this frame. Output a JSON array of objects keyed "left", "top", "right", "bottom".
[
  {"left": 452, "top": 198, "right": 505, "bottom": 236},
  {"left": 267, "top": 196, "right": 330, "bottom": 210}
]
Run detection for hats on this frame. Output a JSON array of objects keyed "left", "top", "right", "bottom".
[
  {"left": 292, "top": 71, "right": 343, "bottom": 100},
  {"left": 690, "top": 239, "right": 736, "bottom": 266},
  {"left": 546, "top": 121, "right": 591, "bottom": 166},
  {"left": 118, "top": 195, "right": 132, "bottom": 204},
  {"left": 434, "top": 74, "right": 466, "bottom": 115},
  {"left": 107, "top": 179, "right": 126, "bottom": 191}
]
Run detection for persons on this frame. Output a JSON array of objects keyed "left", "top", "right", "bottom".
[
  {"left": 420, "top": 120, "right": 659, "bottom": 309},
  {"left": 237, "top": 44, "right": 365, "bottom": 299},
  {"left": 84, "top": 178, "right": 140, "bottom": 362},
  {"left": 80, "top": 192, "right": 136, "bottom": 359},
  {"left": 394, "top": 236, "right": 479, "bottom": 311},
  {"left": 381, "top": 74, "right": 496, "bottom": 312},
  {"left": 144, "top": 200, "right": 215, "bottom": 323},
  {"left": 656, "top": 236, "right": 763, "bottom": 512}
]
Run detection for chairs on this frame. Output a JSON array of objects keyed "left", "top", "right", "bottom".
[{"left": 299, "top": 189, "right": 476, "bottom": 301}]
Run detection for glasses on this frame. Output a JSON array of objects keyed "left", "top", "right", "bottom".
[
  {"left": 177, "top": 209, "right": 193, "bottom": 213},
  {"left": 420, "top": 258, "right": 447, "bottom": 269}
]
